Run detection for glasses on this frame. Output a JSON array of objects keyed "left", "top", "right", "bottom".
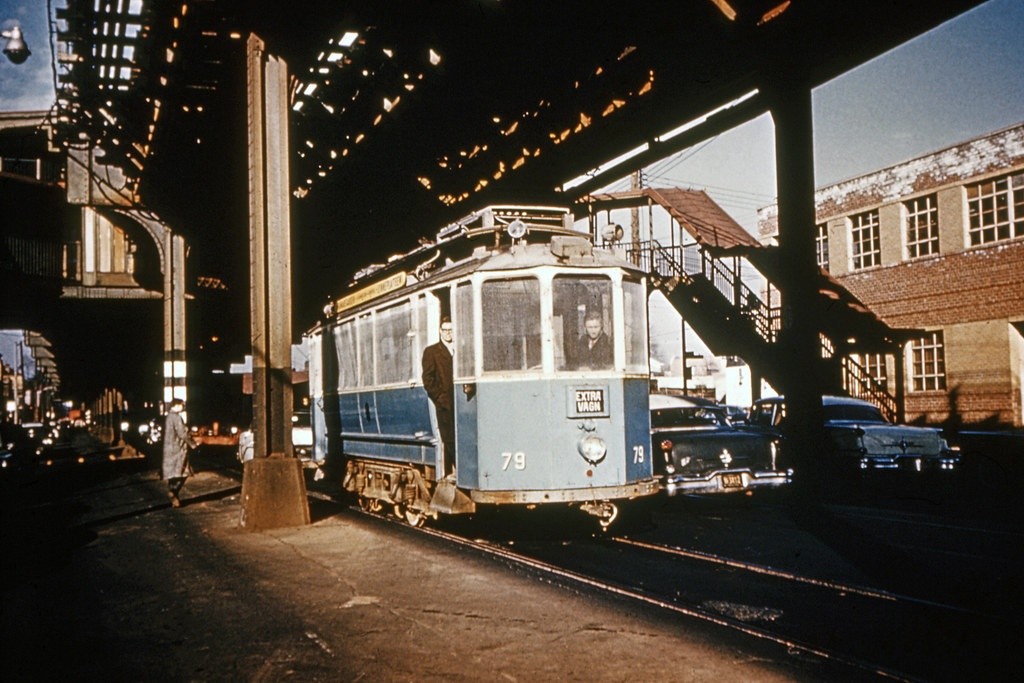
[{"left": 441, "top": 326, "right": 453, "bottom": 333}]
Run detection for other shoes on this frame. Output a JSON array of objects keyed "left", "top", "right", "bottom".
[{"left": 167, "top": 490, "right": 180, "bottom": 508}]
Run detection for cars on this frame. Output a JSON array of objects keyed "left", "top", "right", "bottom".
[
  {"left": 236, "top": 408, "right": 315, "bottom": 474},
  {"left": 720, "top": 403, "right": 751, "bottom": 425}
]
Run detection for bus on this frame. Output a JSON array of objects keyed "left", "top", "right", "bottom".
[{"left": 297, "top": 206, "right": 656, "bottom": 528}]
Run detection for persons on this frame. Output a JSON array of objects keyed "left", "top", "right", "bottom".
[
  {"left": 238, "top": 423, "right": 254, "bottom": 464},
  {"left": 559, "top": 310, "right": 613, "bottom": 372},
  {"left": 421, "top": 314, "right": 456, "bottom": 478},
  {"left": 160, "top": 399, "right": 199, "bottom": 509}
]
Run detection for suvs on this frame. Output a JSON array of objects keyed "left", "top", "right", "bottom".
[
  {"left": 746, "top": 393, "right": 963, "bottom": 515},
  {"left": 647, "top": 393, "right": 794, "bottom": 522}
]
